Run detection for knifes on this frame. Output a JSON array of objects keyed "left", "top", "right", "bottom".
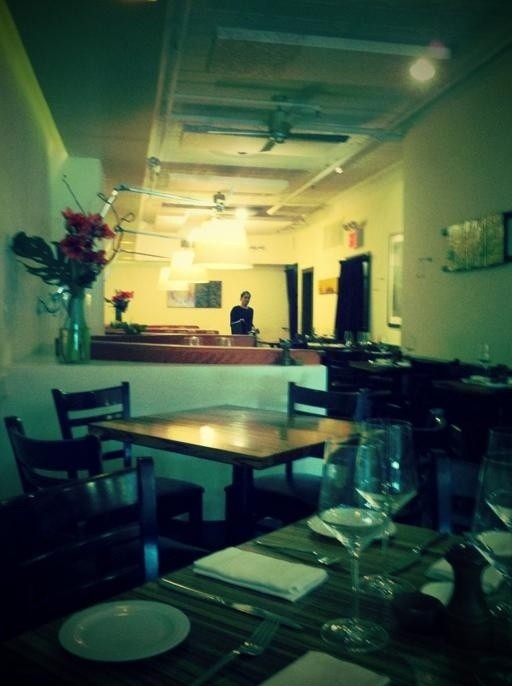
[
  {"left": 157, "top": 577, "right": 304, "bottom": 632},
  {"left": 389, "top": 528, "right": 451, "bottom": 578}
]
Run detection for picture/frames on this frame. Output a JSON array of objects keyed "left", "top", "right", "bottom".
[{"left": 386, "top": 232, "right": 403, "bottom": 328}]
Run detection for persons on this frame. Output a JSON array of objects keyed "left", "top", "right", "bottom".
[{"left": 231, "top": 293, "right": 259, "bottom": 335}]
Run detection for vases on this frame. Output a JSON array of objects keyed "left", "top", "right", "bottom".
[
  {"left": 59, "top": 285, "right": 91, "bottom": 363},
  {"left": 114, "top": 308, "right": 122, "bottom": 322}
]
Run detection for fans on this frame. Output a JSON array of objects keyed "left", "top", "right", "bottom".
[{"left": 112, "top": 109, "right": 351, "bottom": 273}]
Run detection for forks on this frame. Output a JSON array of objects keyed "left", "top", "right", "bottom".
[
  {"left": 254, "top": 538, "right": 348, "bottom": 567},
  {"left": 191, "top": 613, "right": 281, "bottom": 685}
]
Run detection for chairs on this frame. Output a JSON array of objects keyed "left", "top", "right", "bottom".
[
  {"left": 327, "top": 340, "right": 512, "bottom": 503},
  {"left": 221, "top": 379, "right": 361, "bottom": 553},
  {"left": 1, "top": 380, "right": 207, "bottom": 602}
]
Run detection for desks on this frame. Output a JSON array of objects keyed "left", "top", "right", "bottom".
[
  {"left": 0, "top": 503, "right": 512, "bottom": 685},
  {"left": 90, "top": 403, "right": 334, "bottom": 550}
]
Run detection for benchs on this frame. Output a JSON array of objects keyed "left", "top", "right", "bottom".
[{"left": 54, "top": 324, "right": 321, "bottom": 365}]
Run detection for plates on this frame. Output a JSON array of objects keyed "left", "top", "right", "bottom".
[
  {"left": 306, "top": 514, "right": 396, "bottom": 541},
  {"left": 57, "top": 599, "right": 191, "bottom": 663}
]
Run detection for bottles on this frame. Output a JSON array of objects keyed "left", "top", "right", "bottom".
[
  {"left": 59, "top": 296, "right": 90, "bottom": 363},
  {"left": 282, "top": 346, "right": 291, "bottom": 365}
]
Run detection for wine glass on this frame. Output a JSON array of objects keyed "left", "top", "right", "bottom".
[
  {"left": 475, "top": 340, "right": 496, "bottom": 388},
  {"left": 316, "top": 417, "right": 420, "bottom": 653}
]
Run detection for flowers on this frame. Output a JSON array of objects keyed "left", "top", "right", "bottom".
[
  {"left": 11, "top": 204, "right": 117, "bottom": 297},
  {"left": 103, "top": 289, "right": 135, "bottom": 313}
]
